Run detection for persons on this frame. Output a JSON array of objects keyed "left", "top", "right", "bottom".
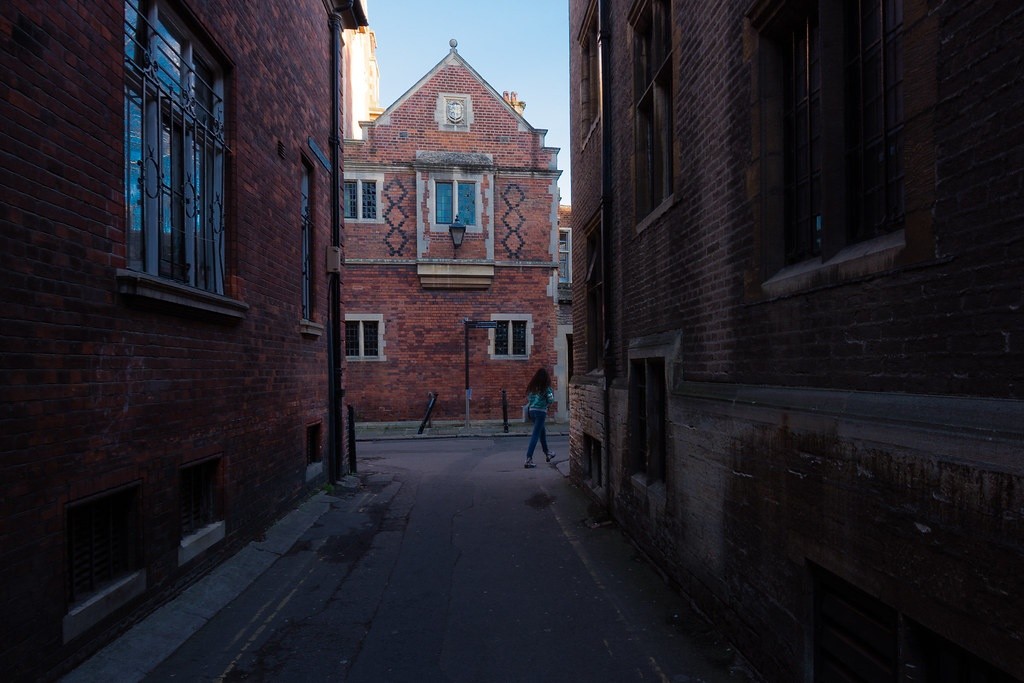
[{"left": 521, "top": 367, "right": 558, "bottom": 470}]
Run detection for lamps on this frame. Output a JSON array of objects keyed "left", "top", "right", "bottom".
[{"left": 449, "top": 215, "right": 466, "bottom": 250}]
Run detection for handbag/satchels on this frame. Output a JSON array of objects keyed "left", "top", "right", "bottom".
[{"left": 522, "top": 403, "right": 530, "bottom": 423}]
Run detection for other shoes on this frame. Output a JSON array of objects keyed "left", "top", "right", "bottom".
[
  {"left": 546, "top": 451, "right": 556, "bottom": 462},
  {"left": 524, "top": 460, "right": 536, "bottom": 468}
]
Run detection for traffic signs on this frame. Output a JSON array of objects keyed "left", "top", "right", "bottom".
[{"left": 466, "top": 320, "right": 498, "bottom": 329}]
